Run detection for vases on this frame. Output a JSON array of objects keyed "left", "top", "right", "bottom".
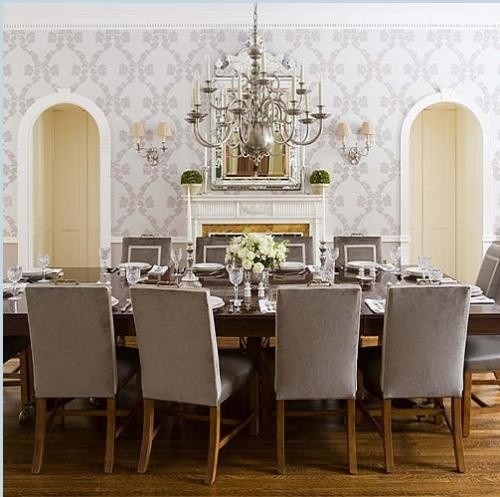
[{"left": 245, "top": 269, "right": 269, "bottom": 283}]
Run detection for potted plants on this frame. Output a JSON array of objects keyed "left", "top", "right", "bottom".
[
  {"left": 309, "top": 169, "right": 331, "bottom": 194},
  {"left": 180, "top": 169, "right": 204, "bottom": 194}
]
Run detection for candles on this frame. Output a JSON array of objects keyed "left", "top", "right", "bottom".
[
  {"left": 192, "top": 86, "right": 196, "bottom": 109},
  {"left": 238, "top": 73, "right": 242, "bottom": 100},
  {"left": 206, "top": 56, "right": 210, "bottom": 79},
  {"left": 195, "top": 77, "right": 199, "bottom": 104},
  {"left": 300, "top": 60, "right": 303, "bottom": 81},
  {"left": 292, "top": 73, "right": 295, "bottom": 100},
  {"left": 261, "top": 45, "right": 264, "bottom": 71},
  {"left": 318, "top": 74, "right": 323, "bottom": 105},
  {"left": 245, "top": 68, "right": 249, "bottom": 87},
  {"left": 305, "top": 84, "right": 309, "bottom": 110}
]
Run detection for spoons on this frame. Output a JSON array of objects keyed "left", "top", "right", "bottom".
[{"left": 234, "top": 299, "right": 241, "bottom": 312}]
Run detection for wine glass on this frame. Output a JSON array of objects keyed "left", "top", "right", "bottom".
[
  {"left": 37, "top": 254, "right": 49, "bottom": 283},
  {"left": 7, "top": 266, "right": 22, "bottom": 300},
  {"left": 100, "top": 248, "right": 111, "bottom": 269},
  {"left": 170, "top": 248, "right": 182, "bottom": 276},
  {"left": 390, "top": 245, "right": 400, "bottom": 271},
  {"left": 126, "top": 268, "right": 140, "bottom": 287},
  {"left": 418, "top": 258, "right": 431, "bottom": 281},
  {"left": 327, "top": 247, "right": 339, "bottom": 274},
  {"left": 228, "top": 268, "right": 243, "bottom": 302}
]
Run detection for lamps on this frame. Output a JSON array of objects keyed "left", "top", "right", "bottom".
[
  {"left": 357, "top": 121, "right": 376, "bottom": 151},
  {"left": 334, "top": 122, "right": 352, "bottom": 152},
  {"left": 130, "top": 123, "right": 146, "bottom": 151},
  {"left": 155, "top": 122, "right": 172, "bottom": 151}
]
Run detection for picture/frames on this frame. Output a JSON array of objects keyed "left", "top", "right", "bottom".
[{"left": 211, "top": 76, "right": 304, "bottom": 192}]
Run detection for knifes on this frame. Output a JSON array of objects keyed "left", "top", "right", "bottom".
[
  {"left": 121, "top": 301, "right": 131, "bottom": 311},
  {"left": 229, "top": 302, "right": 234, "bottom": 312}
]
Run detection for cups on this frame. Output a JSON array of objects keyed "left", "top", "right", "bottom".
[
  {"left": 98, "top": 272, "right": 112, "bottom": 286},
  {"left": 430, "top": 268, "right": 441, "bottom": 279}
]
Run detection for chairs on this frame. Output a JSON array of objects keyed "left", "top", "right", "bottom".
[
  {"left": 361, "top": 284, "right": 472, "bottom": 473},
  {"left": 196, "top": 236, "right": 233, "bottom": 263},
  {"left": 262, "top": 286, "right": 361, "bottom": 474},
  {"left": 334, "top": 236, "right": 382, "bottom": 265},
  {"left": 272, "top": 236, "right": 314, "bottom": 265},
  {"left": 130, "top": 284, "right": 259, "bottom": 484},
  {"left": 474, "top": 244, "right": 500, "bottom": 304},
  {"left": 462, "top": 334, "right": 500, "bottom": 436},
  {"left": 120, "top": 237, "right": 171, "bottom": 266},
  {"left": 25, "top": 283, "right": 139, "bottom": 473}
]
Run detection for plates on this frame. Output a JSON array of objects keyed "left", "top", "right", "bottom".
[
  {"left": 406, "top": 267, "right": 432, "bottom": 277},
  {"left": 119, "top": 262, "right": 152, "bottom": 271},
  {"left": 111, "top": 298, "right": 119, "bottom": 305},
  {"left": 346, "top": 261, "right": 381, "bottom": 270},
  {"left": 279, "top": 261, "right": 307, "bottom": 270},
  {"left": 208, "top": 296, "right": 225, "bottom": 309},
  {"left": 471, "top": 291, "right": 483, "bottom": 295},
  {"left": 194, "top": 263, "right": 221, "bottom": 271}
]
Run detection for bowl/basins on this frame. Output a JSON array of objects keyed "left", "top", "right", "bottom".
[{"left": 471, "top": 286, "right": 481, "bottom": 292}]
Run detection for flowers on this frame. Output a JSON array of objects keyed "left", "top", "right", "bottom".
[{"left": 225, "top": 230, "right": 290, "bottom": 273}]
